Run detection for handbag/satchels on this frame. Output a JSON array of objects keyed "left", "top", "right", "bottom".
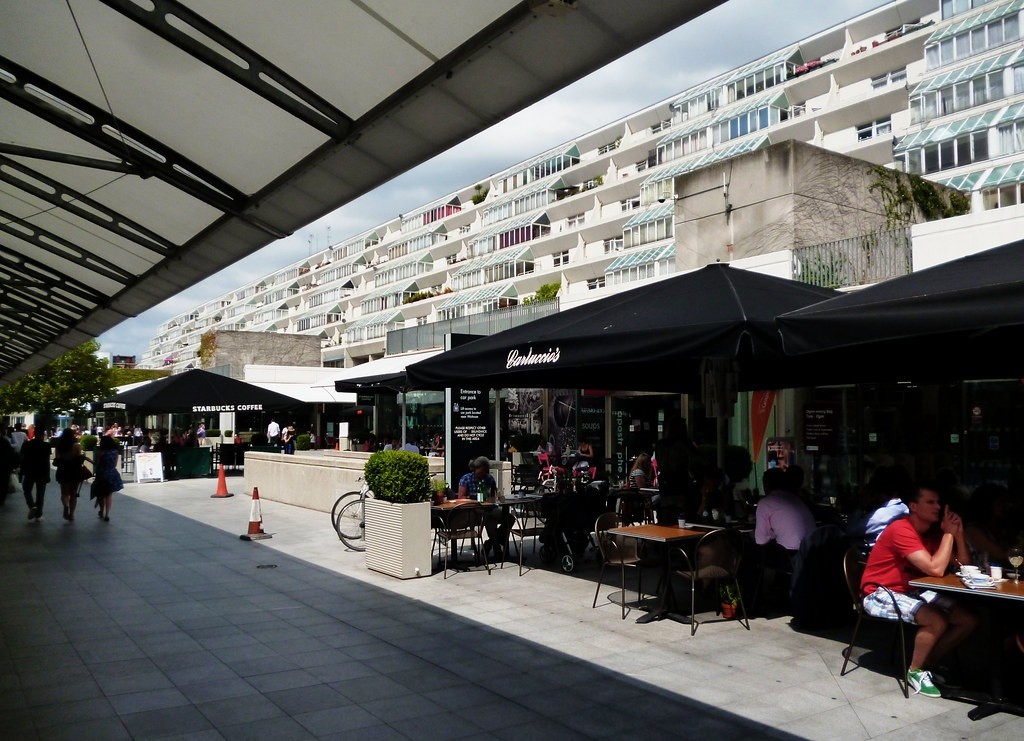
[{"left": 80, "top": 465, "right": 92, "bottom": 480}]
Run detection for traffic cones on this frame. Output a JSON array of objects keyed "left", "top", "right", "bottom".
[
  {"left": 240, "top": 487, "right": 272, "bottom": 542},
  {"left": 210, "top": 463, "right": 235, "bottom": 500}
]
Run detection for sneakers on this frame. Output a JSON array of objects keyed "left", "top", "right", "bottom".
[{"left": 907, "top": 661, "right": 962, "bottom": 697}]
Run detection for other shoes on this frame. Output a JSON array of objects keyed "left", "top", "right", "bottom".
[
  {"left": 28, "top": 506, "right": 38, "bottom": 519},
  {"left": 35, "top": 513, "right": 42, "bottom": 521},
  {"left": 63, "top": 506, "right": 69, "bottom": 520}
]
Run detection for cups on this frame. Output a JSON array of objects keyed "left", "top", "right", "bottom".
[
  {"left": 960, "top": 564, "right": 978, "bottom": 575},
  {"left": 725, "top": 516, "right": 731, "bottom": 523},
  {"left": 830, "top": 496, "right": 836, "bottom": 505},
  {"left": 712, "top": 514, "right": 719, "bottom": 521},
  {"left": 991, "top": 565, "right": 1003, "bottom": 580},
  {"left": 970, "top": 574, "right": 993, "bottom": 586},
  {"left": 678, "top": 519, "right": 685, "bottom": 528}
]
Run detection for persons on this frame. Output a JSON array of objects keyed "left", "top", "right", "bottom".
[
  {"left": 356, "top": 435, "right": 1024, "bottom": 700},
  {"left": 0, "top": 420, "right": 317, "bottom": 523}
]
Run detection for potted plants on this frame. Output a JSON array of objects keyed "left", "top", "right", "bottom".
[
  {"left": 222, "top": 430, "right": 235, "bottom": 444},
  {"left": 364, "top": 449, "right": 433, "bottom": 579},
  {"left": 204, "top": 429, "right": 222, "bottom": 446},
  {"left": 719, "top": 581, "right": 739, "bottom": 619},
  {"left": 80, "top": 435, "right": 98, "bottom": 475},
  {"left": 113, "top": 438, "right": 122, "bottom": 476},
  {"left": 432, "top": 481, "right": 447, "bottom": 507}
]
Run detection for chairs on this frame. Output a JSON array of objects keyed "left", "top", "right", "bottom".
[
  {"left": 842, "top": 546, "right": 912, "bottom": 698},
  {"left": 762, "top": 524, "right": 833, "bottom": 600},
  {"left": 610, "top": 492, "right": 650, "bottom": 525},
  {"left": 431, "top": 501, "right": 489, "bottom": 579},
  {"left": 667, "top": 525, "right": 750, "bottom": 637},
  {"left": 593, "top": 512, "right": 643, "bottom": 619},
  {"left": 501, "top": 500, "right": 539, "bottom": 575},
  {"left": 508, "top": 451, "right": 597, "bottom": 495}
]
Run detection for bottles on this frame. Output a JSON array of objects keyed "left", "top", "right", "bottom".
[
  {"left": 477, "top": 480, "right": 485, "bottom": 503},
  {"left": 443, "top": 495, "right": 447, "bottom": 503},
  {"left": 566, "top": 444, "right": 570, "bottom": 454}
]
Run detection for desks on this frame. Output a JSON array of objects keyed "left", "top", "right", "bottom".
[
  {"left": 607, "top": 523, "right": 707, "bottom": 623},
  {"left": 496, "top": 496, "right": 541, "bottom": 552},
  {"left": 174, "top": 446, "right": 210, "bottom": 477},
  {"left": 908, "top": 560, "right": 1024, "bottom": 721}
]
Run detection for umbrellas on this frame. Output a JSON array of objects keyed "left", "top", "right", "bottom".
[
  {"left": 774, "top": 239, "right": 1024, "bottom": 388},
  {"left": 334, "top": 373, "right": 408, "bottom": 447},
  {"left": 406, "top": 264, "right": 849, "bottom": 448},
  {"left": 91, "top": 368, "right": 309, "bottom": 445}
]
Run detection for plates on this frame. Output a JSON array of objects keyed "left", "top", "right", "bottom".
[
  {"left": 731, "top": 520, "right": 739, "bottom": 523},
  {"left": 1006, "top": 572, "right": 1021, "bottom": 577},
  {"left": 955, "top": 571, "right": 995, "bottom": 587}
]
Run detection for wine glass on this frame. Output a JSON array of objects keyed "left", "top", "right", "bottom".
[{"left": 1009, "top": 548, "right": 1024, "bottom": 587}]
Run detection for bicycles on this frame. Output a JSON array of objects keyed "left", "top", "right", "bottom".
[{"left": 331, "top": 477, "right": 374, "bottom": 552}]
